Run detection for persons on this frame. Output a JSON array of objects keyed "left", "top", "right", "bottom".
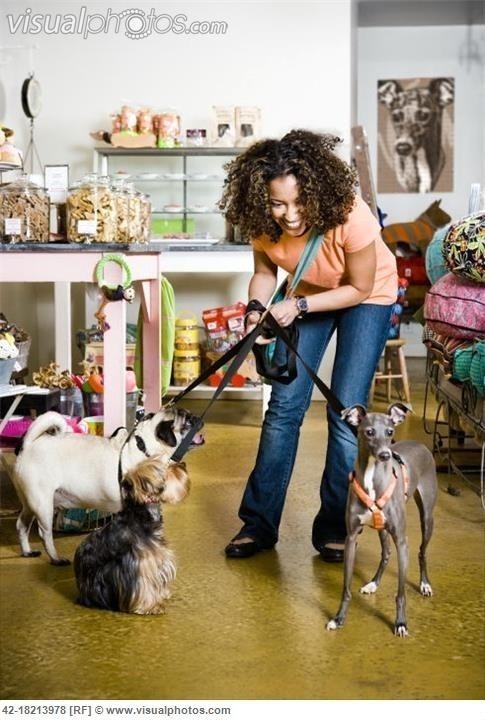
[{"left": 225, "top": 130, "right": 398, "bottom": 564}]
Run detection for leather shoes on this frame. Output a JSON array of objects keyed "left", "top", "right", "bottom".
[
  {"left": 224, "top": 535, "right": 258, "bottom": 558},
  {"left": 321, "top": 543, "right": 344, "bottom": 563}
]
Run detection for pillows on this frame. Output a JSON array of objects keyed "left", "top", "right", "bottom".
[{"left": 425, "top": 207, "right": 485, "bottom": 342}]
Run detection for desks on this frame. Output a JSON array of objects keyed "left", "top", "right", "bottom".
[{"left": 1, "top": 240, "right": 286, "bottom": 437}]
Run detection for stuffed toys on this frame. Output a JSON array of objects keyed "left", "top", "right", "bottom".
[
  {"left": 101, "top": 285, "right": 136, "bottom": 304},
  {"left": 382, "top": 198, "right": 451, "bottom": 260}
]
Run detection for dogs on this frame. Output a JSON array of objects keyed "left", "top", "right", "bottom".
[
  {"left": 325, "top": 399, "right": 440, "bottom": 638},
  {"left": 70, "top": 455, "right": 192, "bottom": 616},
  {"left": 10, "top": 406, "right": 210, "bottom": 566},
  {"left": 377, "top": 77, "right": 454, "bottom": 193}
]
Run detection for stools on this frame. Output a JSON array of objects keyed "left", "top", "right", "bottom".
[{"left": 369, "top": 337, "right": 414, "bottom": 408}]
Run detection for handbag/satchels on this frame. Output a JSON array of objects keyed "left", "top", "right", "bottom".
[{"left": 252, "top": 322, "right": 299, "bottom": 385}]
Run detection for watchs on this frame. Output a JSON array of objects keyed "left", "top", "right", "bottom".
[{"left": 294, "top": 296, "right": 309, "bottom": 319}]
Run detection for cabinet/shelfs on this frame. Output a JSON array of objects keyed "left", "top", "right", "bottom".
[{"left": 94, "top": 143, "right": 258, "bottom": 251}]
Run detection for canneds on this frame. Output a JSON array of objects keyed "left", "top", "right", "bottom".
[{"left": 112, "top": 105, "right": 182, "bottom": 149}]
